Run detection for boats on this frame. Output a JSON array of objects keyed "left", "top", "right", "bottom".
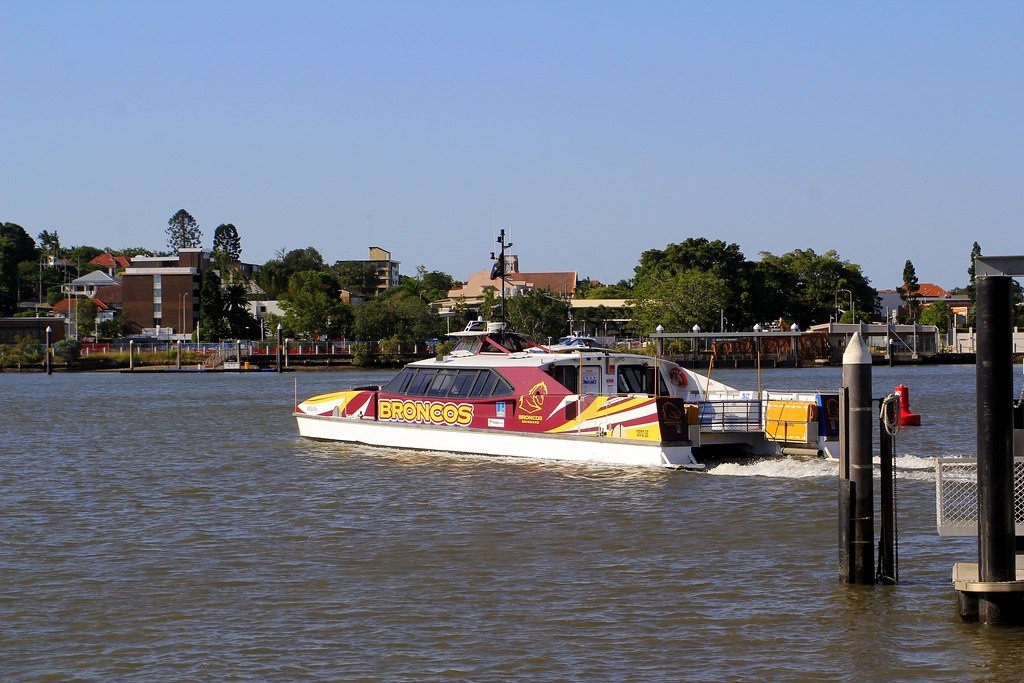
[{"left": 290, "top": 224, "right": 842, "bottom": 479}]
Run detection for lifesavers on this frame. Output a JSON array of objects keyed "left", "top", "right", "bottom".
[{"left": 670, "top": 367, "right": 687, "bottom": 387}]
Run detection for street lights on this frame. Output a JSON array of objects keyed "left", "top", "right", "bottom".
[
  {"left": 419, "top": 289, "right": 426, "bottom": 299},
  {"left": 835, "top": 288, "right": 852, "bottom": 323},
  {"left": 183, "top": 293, "right": 189, "bottom": 345},
  {"left": 720, "top": 308, "right": 728, "bottom": 333}
]
